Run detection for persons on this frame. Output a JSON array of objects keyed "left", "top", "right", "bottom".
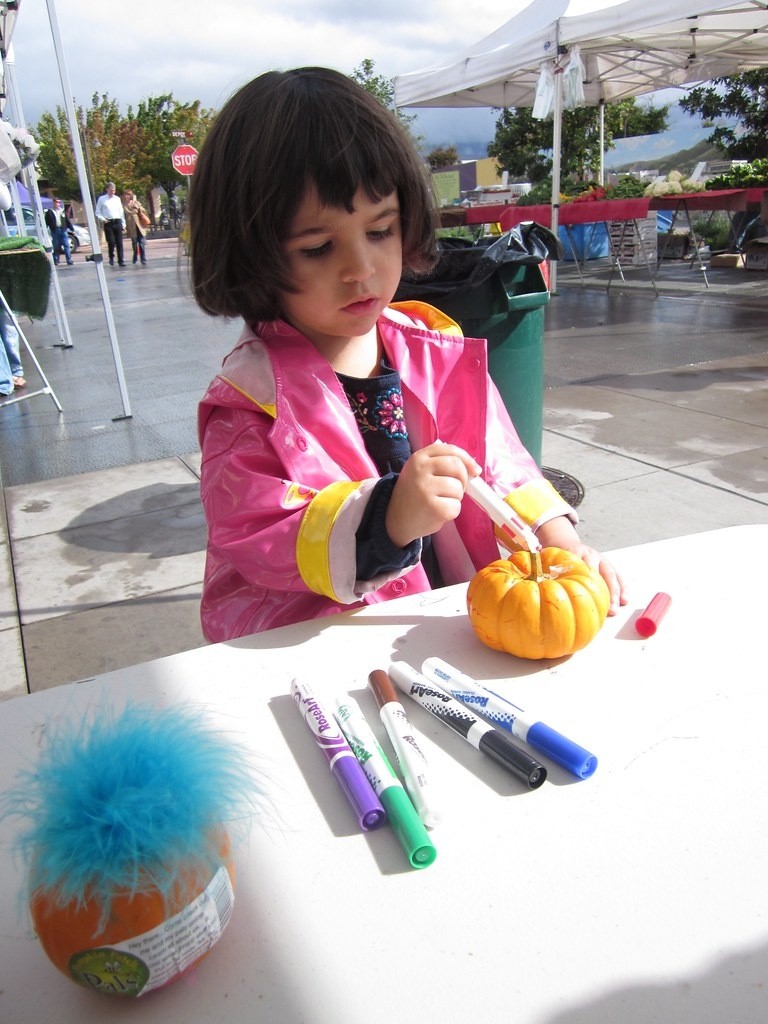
[
  {"left": 175, "top": 67, "right": 629, "bottom": 650},
  {"left": 0, "top": 305, "right": 26, "bottom": 400},
  {"left": 123, "top": 189, "right": 148, "bottom": 264},
  {"left": 45, "top": 197, "right": 76, "bottom": 265},
  {"left": 94, "top": 182, "right": 127, "bottom": 266}
]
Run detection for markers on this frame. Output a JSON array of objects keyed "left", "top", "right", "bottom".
[
  {"left": 392, "top": 661, "right": 548, "bottom": 791},
  {"left": 434, "top": 437, "right": 541, "bottom": 551},
  {"left": 288, "top": 671, "right": 386, "bottom": 832},
  {"left": 420, "top": 655, "right": 598, "bottom": 781},
  {"left": 367, "top": 670, "right": 439, "bottom": 828},
  {"left": 330, "top": 693, "right": 437, "bottom": 870}
]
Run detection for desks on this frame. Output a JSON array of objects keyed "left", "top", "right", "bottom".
[
  {"left": 0, "top": 524, "right": 768, "bottom": 1024},
  {"left": 499, "top": 189, "right": 749, "bottom": 296},
  {"left": 691, "top": 187, "right": 768, "bottom": 272},
  {"left": 435, "top": 204, "right": 517, "bottom": 240},
  {"left": 0, "top": 237, "right": 64, "bottom": 416}
]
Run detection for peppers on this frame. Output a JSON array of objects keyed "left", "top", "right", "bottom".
[{"left": 601, "top": 175, "right": 652, "bottom": 200}]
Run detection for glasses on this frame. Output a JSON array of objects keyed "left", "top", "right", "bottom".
[{"left": 55, "top": 202, "right": 60, "bottom": 203}]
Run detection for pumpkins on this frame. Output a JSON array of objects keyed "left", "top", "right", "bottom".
[
  {"left": 466, "top": 547, "right": 610, "bottom": 659},
  {"left": 28, "top": 816, "right": 236, "bottom": 995}
]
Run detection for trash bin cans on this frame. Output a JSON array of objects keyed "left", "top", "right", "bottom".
[{"left": 389, "top": 238, "right": 550, "bottom": 466}]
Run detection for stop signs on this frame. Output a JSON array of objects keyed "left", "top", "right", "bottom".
[{"left": 170, "top": 144, "right": 201, "bottom": 176}]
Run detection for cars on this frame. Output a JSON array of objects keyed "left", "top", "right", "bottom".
[{"left": 3, "top": 204, "right": 92, "bottom": 253}]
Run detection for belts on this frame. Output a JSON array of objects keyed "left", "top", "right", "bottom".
[{"left": 56, "top": 227, "right": 62, "bottom": 228}]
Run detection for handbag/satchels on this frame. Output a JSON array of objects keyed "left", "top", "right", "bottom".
[{"left": 139, "top": 212, "right": 151, "bottom": 224}]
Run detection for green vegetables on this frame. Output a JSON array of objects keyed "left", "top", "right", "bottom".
[{"left": 705, "top": 158, "right": 768, "bottom": 190}]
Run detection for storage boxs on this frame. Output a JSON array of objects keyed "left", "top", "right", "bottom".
[
  {"left": 461, "top": 190, "right": 511, "bottom": 203},
  {"left": 657, "top": 211, "right": 673, "bottom": 234},
  {"left": 746, "top": 237, "right": 768, "bottom": 270},
  {"left": 558, "top": 220, "right": 610, "bottom": 260},
  {"left": 710, "top": 253, "right": 746, "bottom": 267},
  {"left": 609, "top": 210, "right": 658, "bottom": 264}
]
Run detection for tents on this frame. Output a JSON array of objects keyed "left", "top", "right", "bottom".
[
  {"left": 0, "top": 181, "right": 66, "bottom": 213},
  {"left": 394, "top": 0, "right": 768, "bottom": 299}
]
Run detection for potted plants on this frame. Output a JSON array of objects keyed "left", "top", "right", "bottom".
[{"left": 657, "top": 233, "right": 690, "bottom": 258}]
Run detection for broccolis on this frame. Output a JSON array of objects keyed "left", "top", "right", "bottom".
[
  {"left": 516, "top": 176, "right": 599, "bottom": 206},
  {"left": 643, "top": 170, "right": 704, "bottom": 197}
]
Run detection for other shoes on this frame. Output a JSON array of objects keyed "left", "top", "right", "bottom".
[
  {"left": 141, "top": 261, "right": 145, "bottom": 264},
  {"left": 68, "top": 262, "right": 74, "bottom": 265},
  {"left": 133, "top": 260, "right": 136, "bottom": 264},
  {"left": 55, "top": 261, "right": 58, "bottom": 265},
  {"left": 109, "top": 256, "right": 114, "bottom": 265},
  {"left": 119, "top": 264, "right": 126, "bottom": 266}
]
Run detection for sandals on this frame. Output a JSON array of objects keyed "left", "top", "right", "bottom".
[{"left": 13, "top": 376, "right": 26, "bottom": 388}]
